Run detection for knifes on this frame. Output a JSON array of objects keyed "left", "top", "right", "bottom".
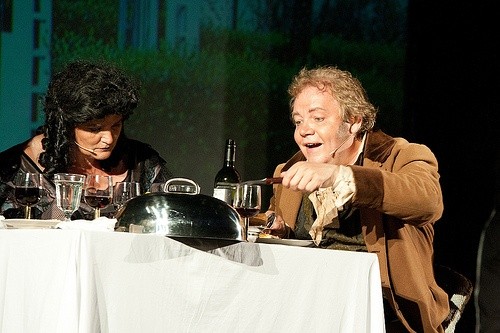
[{"left": 241, "top": 176, "right": 283, "bottom": 185}]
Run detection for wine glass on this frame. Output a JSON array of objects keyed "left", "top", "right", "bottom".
[
  {"left": 14, "top": 172, "right": 44, "bottom": 219},
  {"left": 232, "top": 185, "right": 261, "bottom": 241},
  {"left": 83, "top": 175, "right": 114, "bottom": 219},
  {"left": 54, "top": 173, "right": 86, "bottom": 224}
]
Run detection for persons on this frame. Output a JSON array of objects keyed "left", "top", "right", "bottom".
[
  {"left": 266, "top": 72, "right": 449, "bottom": 333},
  {"left": 0, "top": 60, "right": 174, "bottom": 221}
]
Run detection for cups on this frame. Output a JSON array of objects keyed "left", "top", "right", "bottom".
[
  {"left": 112, "top": 181, "right": 140, "bottom": 206},
  {"left": 150, "top": 183, "right": 194, "bottom": 193}
]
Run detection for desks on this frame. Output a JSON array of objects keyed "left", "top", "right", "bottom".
[{"left": 0, "top": 228, "right": 387, "bottom": 333}]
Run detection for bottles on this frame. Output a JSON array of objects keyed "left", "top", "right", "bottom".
[{"left": 211, "top": 138, "right": 241, "bottom": 208}]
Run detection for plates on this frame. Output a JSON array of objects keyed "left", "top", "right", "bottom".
[
  {"left": 256, "top": 237, "right": 314, "bottom": 247},
  {"left": 4, "top": 218, "right": 60, "bottom": 228}
]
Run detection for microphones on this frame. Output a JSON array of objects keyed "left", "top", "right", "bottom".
[
  {"left": 74, "top": 141, "right": 94, "bottom": 152},
  {"left": 329, "top": 133, "right": 354, "bottom": 155}
]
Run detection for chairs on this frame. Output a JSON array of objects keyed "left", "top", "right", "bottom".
[{"left": 434, "top": 268, "right": 473, "bottom": 333}]
